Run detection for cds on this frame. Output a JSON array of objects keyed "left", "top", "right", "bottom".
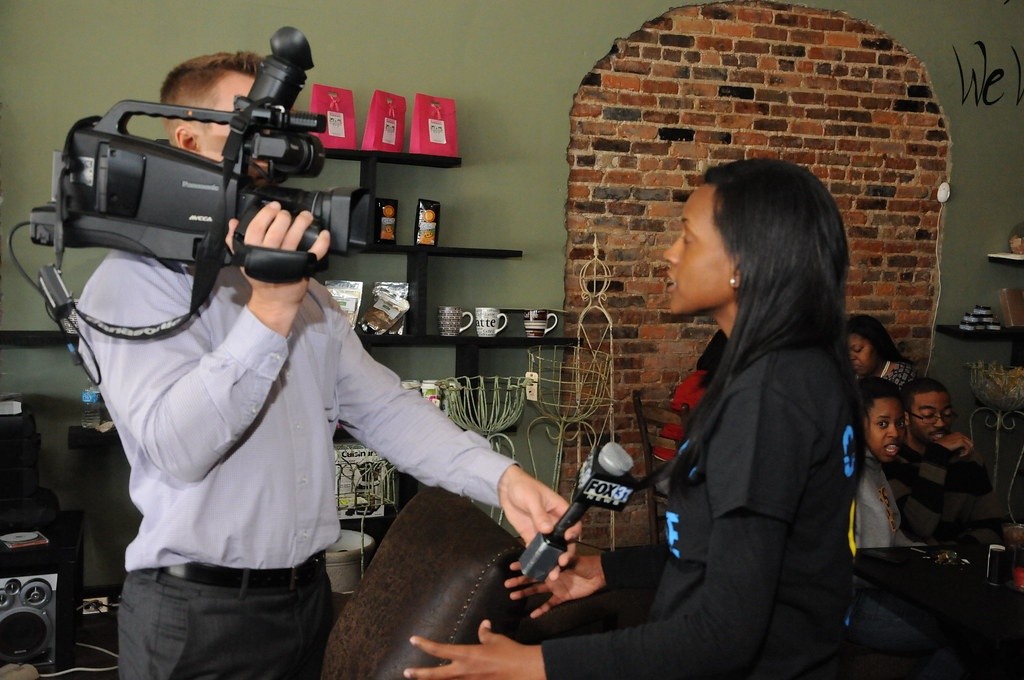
[{"left": 0, "top": 532, "right": 38, "bottom": 542}]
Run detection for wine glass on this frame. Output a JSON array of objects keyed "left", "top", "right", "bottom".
[{"left": 1003, "top": 524, "right": 1024, "bottom": 591}]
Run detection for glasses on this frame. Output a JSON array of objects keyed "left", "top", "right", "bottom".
[{"left": 906, "top": 409, "right": 959, "bottom": 424}]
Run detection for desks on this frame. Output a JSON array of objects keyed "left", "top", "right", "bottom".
[
  {"left": 853, "top": 542, "right": 1024, "bottom": 680},
  {"left": 0, "top": 509, "right": 84, "bottom": 673},
  {"left": 331, "top": 536, "right": 642, "bottom": 644}
]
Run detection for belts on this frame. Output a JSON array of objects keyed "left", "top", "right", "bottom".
[{"left": 159, "top": 549, "right": 326, "bottom": 590}]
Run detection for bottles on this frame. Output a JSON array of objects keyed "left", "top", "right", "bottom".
[{"left": 81, "top": 376, "right": 100, "bottom": 429}]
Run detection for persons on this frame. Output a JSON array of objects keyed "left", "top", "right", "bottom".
[
  {"left": 813, "top": 311, "right": 1024, "bottom": 680},
  {"left": 406, "top": 155, "right": 863, "bottom": 679},
  {"left": 63, "top": 48, "right": 582, "bottom": 680}
]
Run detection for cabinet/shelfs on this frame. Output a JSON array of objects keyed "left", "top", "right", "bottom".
[
  {"left": 67, "top": 147, "right": 583, "bottom": 551},
  {"left": 935, "top": 256, "right": 1024, "bottom": 413}
]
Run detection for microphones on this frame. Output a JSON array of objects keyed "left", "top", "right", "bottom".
[{"left": 519, "top": 442, "right": 638, "bottom": 583}]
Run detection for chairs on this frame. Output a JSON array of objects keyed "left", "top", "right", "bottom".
[
  {"left": 320, "top": 486, "right": 531, "bottom": 680},
  {"left": 632, "top": 394, "right": 692, "bottom": 545}
]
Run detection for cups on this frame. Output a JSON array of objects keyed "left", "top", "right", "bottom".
[
  {"left": 402, "top": 380, "right": 459, "bottom": 413},
  {"left": 524, "top": 309, "right": 557, "bottom": 337},
  {"left": 475, "top": 307, "right": 508, "bottom": 336},
  {"left": 438, "top": 306, "right": 473, "bottom": 336}
]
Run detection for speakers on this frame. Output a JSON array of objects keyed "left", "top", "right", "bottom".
[{"left": 0, "top": 546, "right": 74, "bottom": 668}]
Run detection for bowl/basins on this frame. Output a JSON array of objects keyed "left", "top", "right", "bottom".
[{"left": 323, "top": 530, "right": 374, "bottom": 595}]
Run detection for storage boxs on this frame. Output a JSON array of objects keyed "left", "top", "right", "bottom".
[{"left": 333, "top": 443, "right": 384, "bottom": 519}]
